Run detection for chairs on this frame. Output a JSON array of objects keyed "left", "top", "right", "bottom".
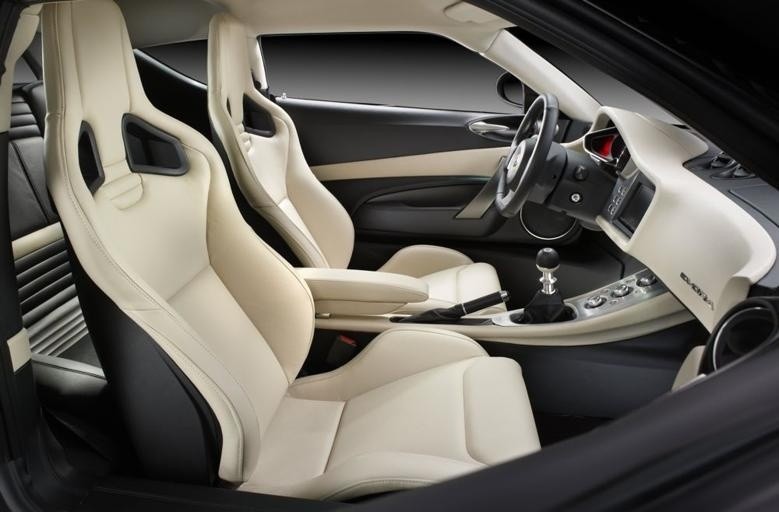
[
  {"left": 41, "top": 0, "right": 543, "bottom": 501},
  {"left": 206, "top": 9, "right": 508, "bottom": 319},
  {"left": 7, "top": 54, "right": 107, "bottom": 399}
]
